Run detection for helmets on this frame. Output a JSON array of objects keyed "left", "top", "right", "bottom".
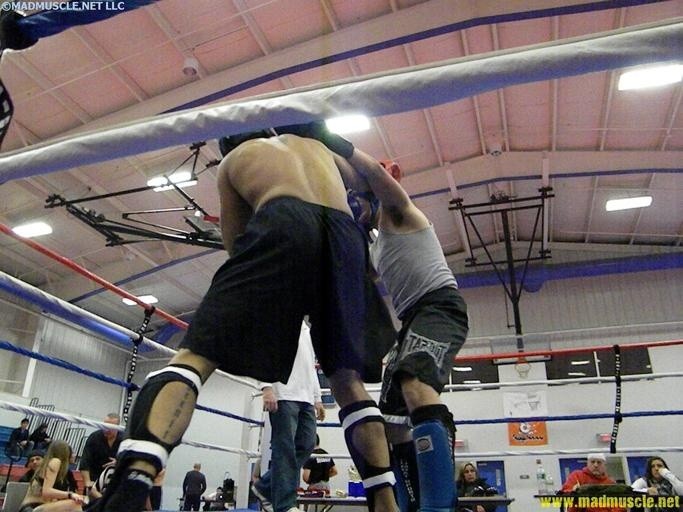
[
  {"left": 348, "top": 190, "right": 379, "bottom": 230},
  {"left": 219, "top": 127, "right": 276, "bottom": 157}
]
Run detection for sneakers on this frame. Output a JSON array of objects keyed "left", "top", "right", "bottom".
[{"left": 251, "top": 485, "right": 274, "bottom": 512}]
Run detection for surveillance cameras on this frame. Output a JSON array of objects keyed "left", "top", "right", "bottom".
[{"left": 182, "top": 58, "right": 197, "bottom": 77}]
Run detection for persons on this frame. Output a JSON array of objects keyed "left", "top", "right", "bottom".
[
  {"left": 6, "top": 413, "right": 126, "bottom": 512},
  {"left": 300, "top": 118, "right": 469, "bottom": 512},
  {"left": 630, "top": 457, "right": 683, "bottom": 512},
  {"left": 250, "top": 458, "right": 261, "bottom": 511},
  {"left": 454, "top": 463, "right": 498, "bottom": 512},
  {"left": 85, "top": 133, "right": 404, "bottom": 511},
  {"left": 182, "top": 464, "right": 207, "bottom": 512},
  {"left": 558, "top": 453, "right": 628, "bottom": 512},
  {"left": 150, "top": 469, "right": 168, "bottom": 511},
  {"left": 302, "top": 434, "right": 338, "bottom": 495}
]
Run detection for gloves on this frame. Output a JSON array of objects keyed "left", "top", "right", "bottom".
[
  {"left": 380, "top": 160, "right": 401, "bottom": 183},
  {"left": 274, "top": 120, "right": 354, "bottom": 158}
]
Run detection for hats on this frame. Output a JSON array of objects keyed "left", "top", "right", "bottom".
[{"left": 587, "top": 453, "right": 606, "bottom": 462}]
